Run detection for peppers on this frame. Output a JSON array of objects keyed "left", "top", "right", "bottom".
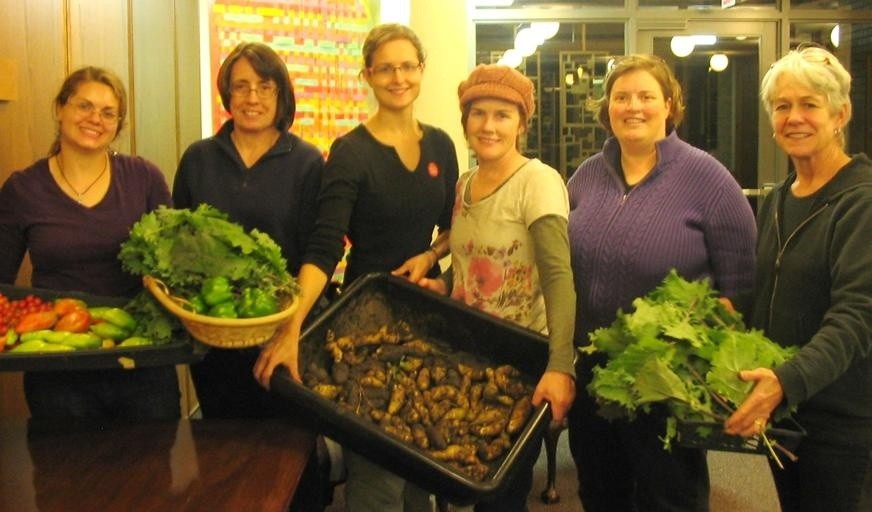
[{"left": 301, "top": 317, "right": 534, "bottom": 480}]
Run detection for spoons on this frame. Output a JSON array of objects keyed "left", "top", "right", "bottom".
[{"left": 0, "top": 294, "right": 53, "bottom": 334}]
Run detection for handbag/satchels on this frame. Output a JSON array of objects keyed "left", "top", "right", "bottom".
[
  {"left": 424, "top": 248, "right": 434, "bottom": 269},
  {"left": 429, "top": 243, "right": 440, "bottom": 260}
]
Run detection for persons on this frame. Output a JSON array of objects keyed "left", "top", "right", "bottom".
[
  {"left": 0, "top": 60, "right": 183, "bottom": 426},
  {"left": 156, "top": 41, "right": 331, "bottom": 428},
  {"left": 248, "top": 21, "right": 464, "bottom": 511},
  {"left": 413, "top": 58, "right": 579, "bottom": 511},
  {"left": 709, "top": 40, "right": 871, "bottom": 510},
  {"left": 554, "top": 54, "right": 760, "bottom": 510}
]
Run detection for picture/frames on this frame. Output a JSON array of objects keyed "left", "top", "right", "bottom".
[{"left": 1, "top": 419, "right": 318, "bottom": 511}]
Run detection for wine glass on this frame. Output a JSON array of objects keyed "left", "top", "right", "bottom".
[{"left": 754, "top": 419, "right": 768, "bottom": 430}]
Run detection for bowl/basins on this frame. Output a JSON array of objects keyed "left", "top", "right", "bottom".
[{"left": 51, "top": 148, "right": 115, "bottom": 208}]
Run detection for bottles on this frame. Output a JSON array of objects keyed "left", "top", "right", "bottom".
[{"left": 458, "top": 64, "right": 536, "bottom": 123}]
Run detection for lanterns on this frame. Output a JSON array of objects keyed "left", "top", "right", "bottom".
[{"left": 6, "top": 298, "right": 151, "bottom": 352}]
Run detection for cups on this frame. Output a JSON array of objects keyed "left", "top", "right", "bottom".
[
  {"left": 230, "top": 82, "right": 279, "bottom": 98},
  {"left": 369, "top": 61, "right": 420, "bottom": 75},
  {"left": 66, "top": 102, "right": 122, "bottom": 124},
  {"left": 770, "top": 54, "right": 830, "bottom": 69}
]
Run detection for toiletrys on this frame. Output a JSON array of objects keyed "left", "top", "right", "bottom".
[{"left": 183, "top": 277, "right": 279, "bottom": 319}]
